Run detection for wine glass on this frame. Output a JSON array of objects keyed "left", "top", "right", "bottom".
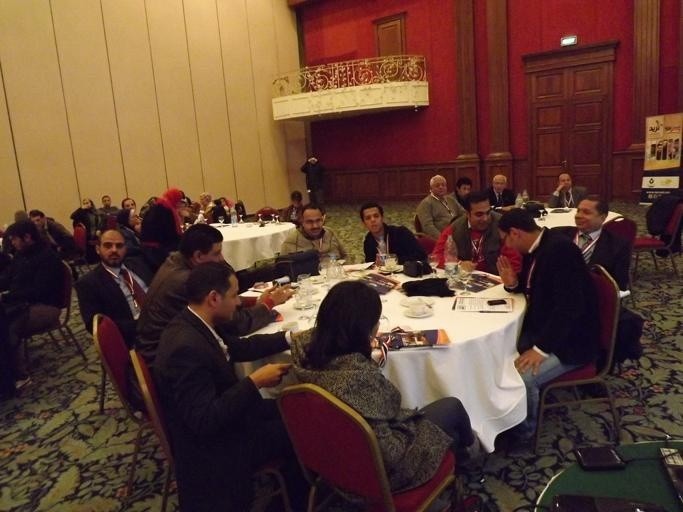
[
  {"left": 537, "top": 210, "right": 545, "bottom": 222},
  {"left": 278, "top": 253, "right": 398, "bottom": 331},
  {"left": 427, "top": 254, "right": 439, "bottom": 278},
  {"left": 180, "top": 207, "right": 280, "bottom": 234},
  {"left": 457, "top": 261, "right": 472, "bottom": 297}
]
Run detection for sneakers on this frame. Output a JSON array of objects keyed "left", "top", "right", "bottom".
[
  {"left": 506, "top": 435, "right": 533, "bottom": 456},
  {"left": 14, "top": 375, "right": 32, "bottom": 390}
]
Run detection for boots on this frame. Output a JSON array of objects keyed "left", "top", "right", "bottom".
[{"left": 453, "top": 430, "right": 486, "bottom": 486}]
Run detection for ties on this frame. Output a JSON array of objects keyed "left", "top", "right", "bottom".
[
  {"left": 498, "top": 193, "right": 502, "bottom": 206},
  {"left": 565, "top": 191, "right": 573, "bottom": 208},
  {"left": 119, "top": 268, "right": 147, "bottom": 313}
]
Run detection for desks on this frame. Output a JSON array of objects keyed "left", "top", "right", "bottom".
[
  {"left": 214, "top": 222, "right": 316, "bottom": 273},
  {"left": 236, "top": 260, "right": 528, "bottom": 453}
]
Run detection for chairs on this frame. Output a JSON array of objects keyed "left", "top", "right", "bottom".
[
  {"left": 21, "top": 213, "right": 118, "bottom": 372},
  {"left": 93, "top": 312, "right": 462, "bottom": 511},
  {"left": 412, "top": 195, "right": 680, "bottom": 305},
  {"left": 527, "top": 264, "right": 623, "bottom": 457},
  {"left": 205, "top": 201, "right": 297, "bottom": 225}
]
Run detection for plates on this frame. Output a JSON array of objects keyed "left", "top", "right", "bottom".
[
  {"left": 401, "top": 296, "right": 437, "bottom": 305},
  {"left": 403, "top": 309, "right": 432, "bottom": 318}
]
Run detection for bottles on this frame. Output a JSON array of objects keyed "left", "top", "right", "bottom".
[
  {"left": 514, "top": 193, "right": 523, "bottom": 206},
  {"left": 521, "top": 190, "right": 528, "bottom": 204},
  {"left": 444, "top": 235, "right": 458, "bottom": 278}
]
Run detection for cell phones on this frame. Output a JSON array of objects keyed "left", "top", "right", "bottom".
[
  {"left": 487, "top": 300, "right": 506, "bottom": 305},
  {"left": 574, "top": 446, "right": 625, "bottom": 470}
]
